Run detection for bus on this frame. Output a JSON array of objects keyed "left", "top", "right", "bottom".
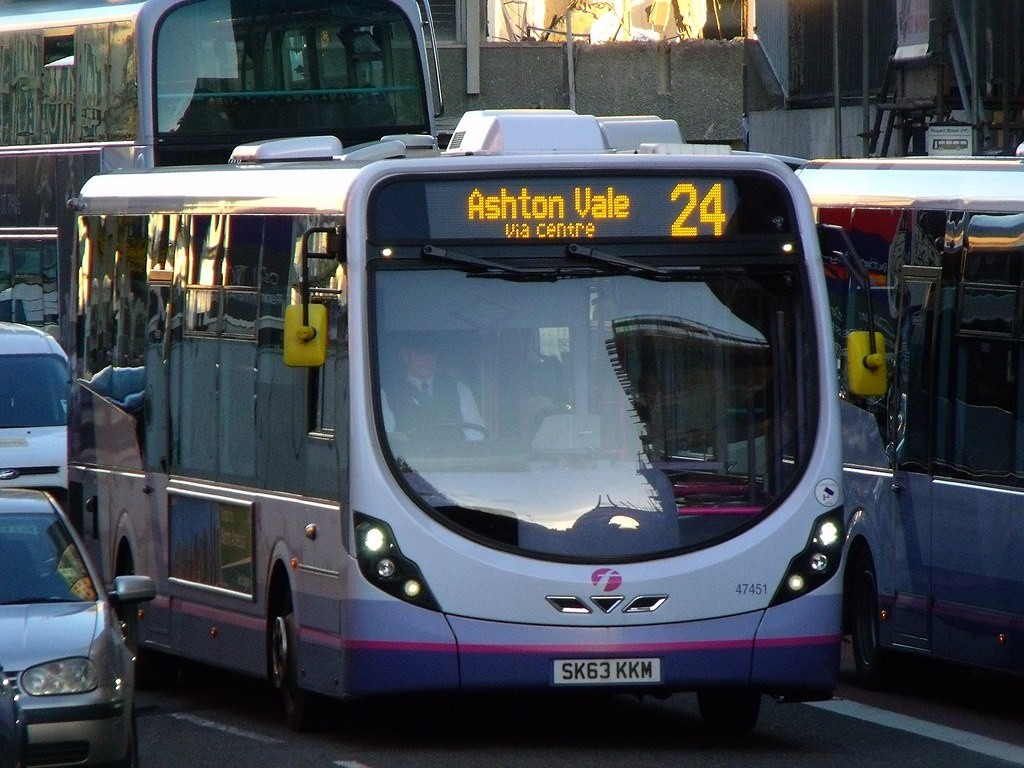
[
  {"left": 793, "top": 158, "right": 1024, "bottom": 694},
  {"left": 0, "top": 0, "right": 888, "bottom": 740}
]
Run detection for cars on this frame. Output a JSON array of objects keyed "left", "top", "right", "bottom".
[{"left": 0, "top": 489, "right": 155, "bottom": 768}]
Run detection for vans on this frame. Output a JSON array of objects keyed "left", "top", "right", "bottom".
[{"left": 0, "top": 322, "right": 69, "bottom": 492}]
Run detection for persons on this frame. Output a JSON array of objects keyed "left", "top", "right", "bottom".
[{"left": 379, "top": 332, "right": 488, "bottom": 455}]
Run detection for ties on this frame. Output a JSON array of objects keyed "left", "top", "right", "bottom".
[{"left": 421, "top": 381, "right": 429, "bottom": 394}]
